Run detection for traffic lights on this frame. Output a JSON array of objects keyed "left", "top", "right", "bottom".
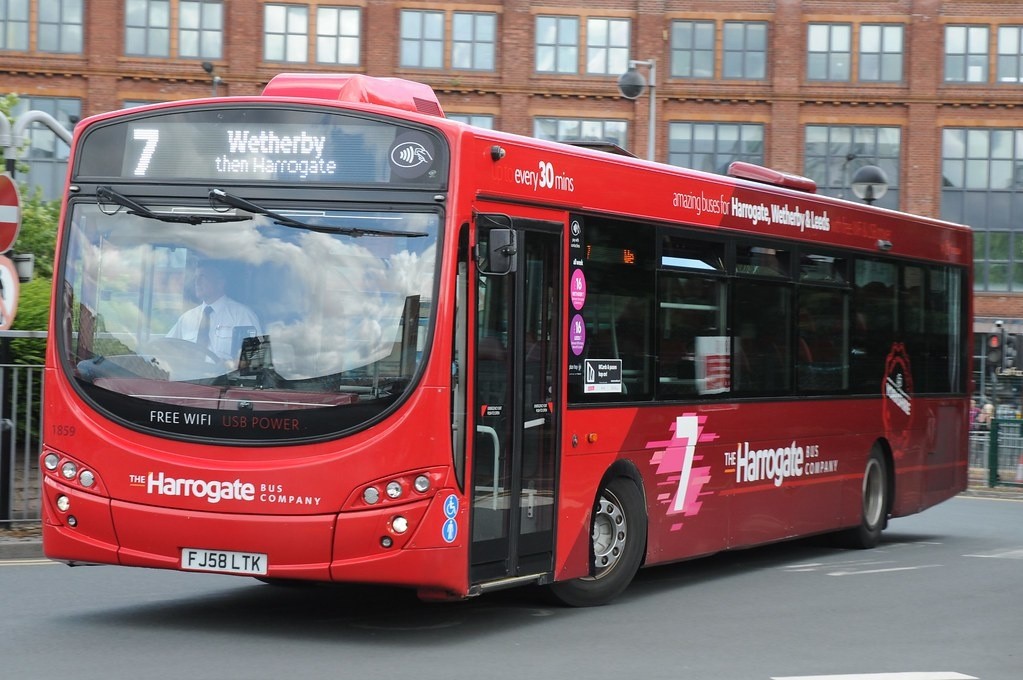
[{"left": 986, "top": 334, "right": 1002, "bottom": 363}]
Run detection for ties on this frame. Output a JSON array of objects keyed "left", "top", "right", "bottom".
[{"left": 195, "top": 305, "right": 214, "bottom": 361}]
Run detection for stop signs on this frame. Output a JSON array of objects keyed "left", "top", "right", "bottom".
[{"left": 0, "top": 173, "right": 21, "bottom": 252}]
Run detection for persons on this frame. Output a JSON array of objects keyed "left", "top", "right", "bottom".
[
  {"left": 969, "top": 400, "right": 994, "bottom": 468},
  {"left": 164, "top": 259, "right": 262, "bottom": 365}
]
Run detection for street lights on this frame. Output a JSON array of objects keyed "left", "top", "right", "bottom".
[{"left": 617, "top": 59, "right": 657, "bottom": 161}]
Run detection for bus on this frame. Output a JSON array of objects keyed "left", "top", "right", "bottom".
[{"left": 32, "top": 70, "right": 975, "bottom": 615}]
